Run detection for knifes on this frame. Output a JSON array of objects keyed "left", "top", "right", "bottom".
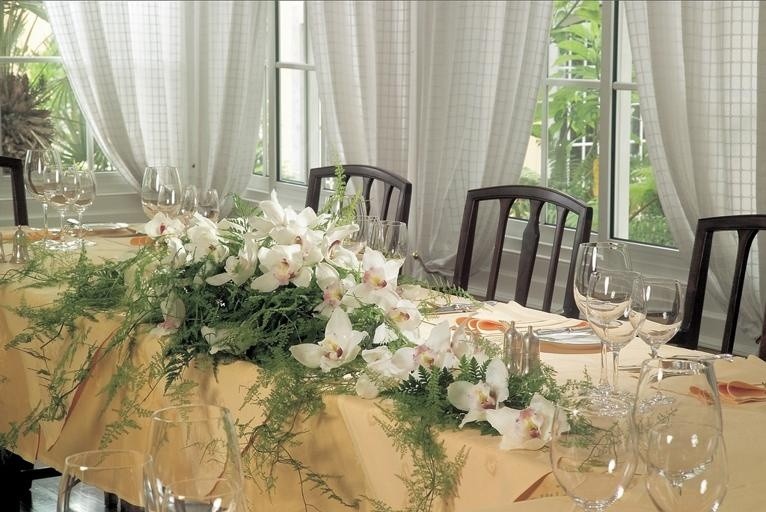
[
  {"left": 535, "top": 325, "right": 592, "bottom": 336},
  {"left": 417, "top": 300, "right": 498, "bottom": 316}
]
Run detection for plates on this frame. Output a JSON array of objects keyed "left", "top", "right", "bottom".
[{"left": 532, "top": 329, "right": 605, "bottom": 346}]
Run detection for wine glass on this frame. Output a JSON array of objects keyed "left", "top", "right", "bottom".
[
  {"left": 23, "top": 147, "right": 96, "bottom": 252},
  {"left": 550, "top": 241, "right": 728, "bottom": 511}
]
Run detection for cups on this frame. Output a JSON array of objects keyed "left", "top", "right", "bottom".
[
  {"left": 49, "top": 403, "right": 249, "bottom": 511},
  {"left": 139, "top": 164, "right": 221, "bottom": 225},
  {"left": 327, "top": 192, "right": 409, "bottom": 273}
]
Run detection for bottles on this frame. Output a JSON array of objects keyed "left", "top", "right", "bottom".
[
  {"left": 523, "top": 324, "right": 541, "bottom": 378},
  {"left": 501, "top": 321, "right": 523, "bottom": 376},
  {"left": 0, "top": 232, "right": 6, "bottom": 264},
  {"left": 9, "top": 223, "right": 30, "bottom": 264}
]
